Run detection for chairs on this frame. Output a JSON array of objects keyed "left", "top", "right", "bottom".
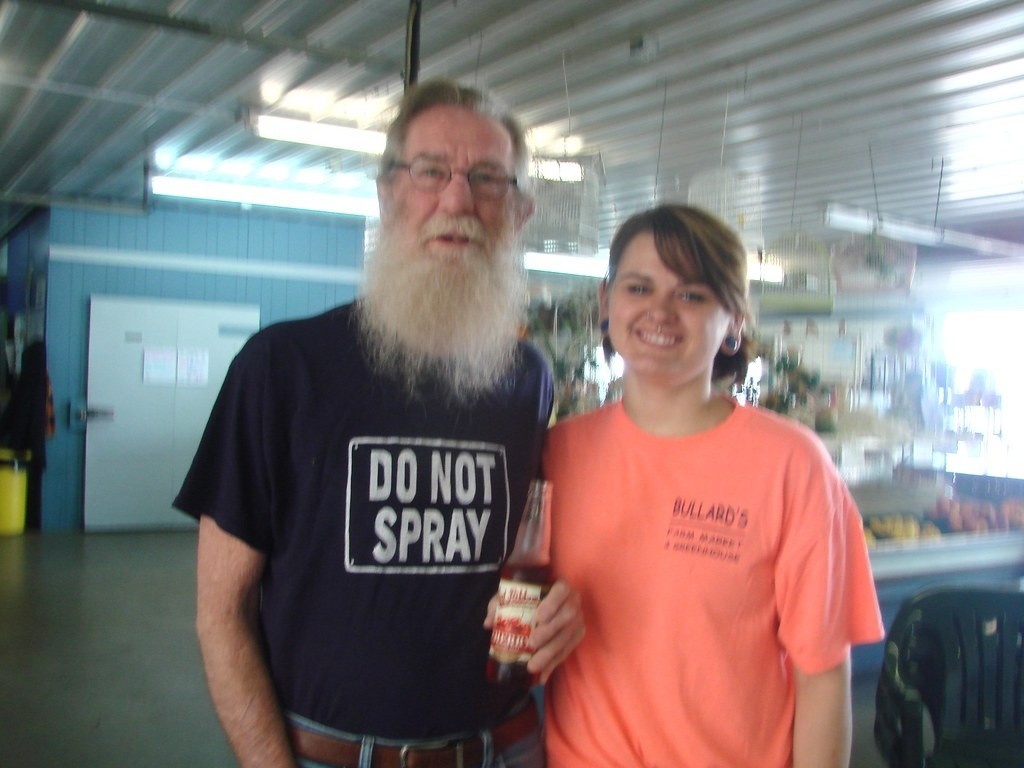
[{"left": 872, "top": 586, "right": 1024, "bottom": 768}]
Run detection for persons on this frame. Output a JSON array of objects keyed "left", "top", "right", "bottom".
[
  {"left": 172, "top": 79, "right": 585, "bottom": 768},
  {"left": 539, "top": 205, "right": 888, "bottom": 768}
]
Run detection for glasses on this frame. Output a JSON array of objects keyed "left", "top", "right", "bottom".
[{"left": 392, "top": 156, "right": 517, "bottom": 198}]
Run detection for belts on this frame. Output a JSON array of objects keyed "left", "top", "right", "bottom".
[{"left": 288, "top": 698, "right": 538, "bottom": 768}]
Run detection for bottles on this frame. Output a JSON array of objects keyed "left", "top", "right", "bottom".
[{"left": 484, "top": 477, "right": 554, "bottom": 690}]
